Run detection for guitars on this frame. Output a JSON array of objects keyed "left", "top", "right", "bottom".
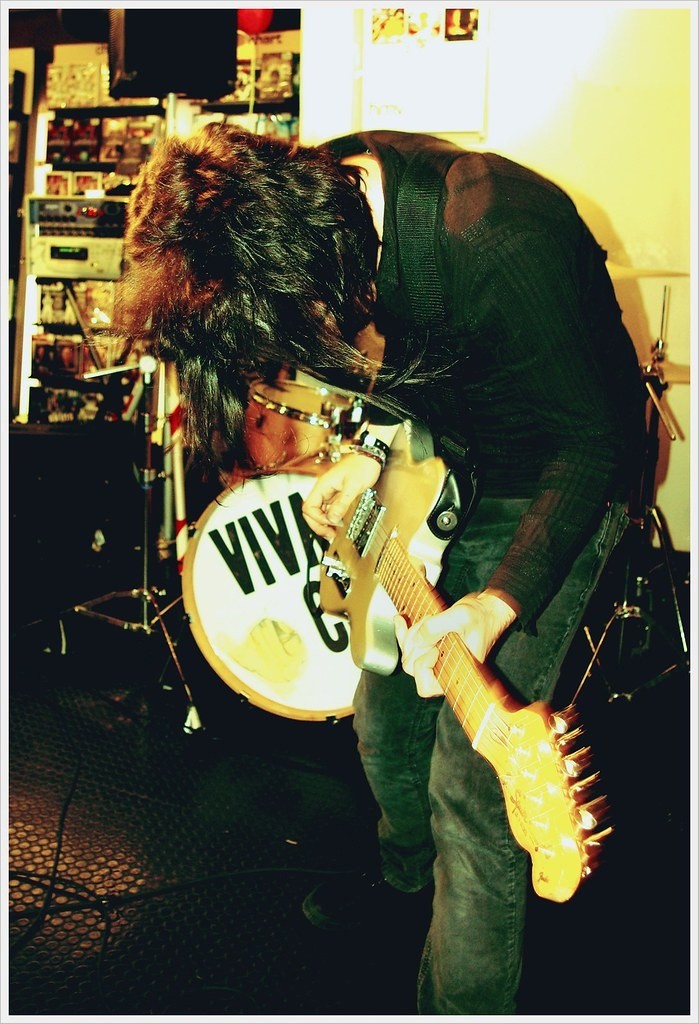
[{"left": 318, "top": 422, "right": 612, "bottom": 903}]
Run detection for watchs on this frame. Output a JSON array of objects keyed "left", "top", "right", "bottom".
[{"left": 358, "top": 431, "right": 390, "bottom": 457}]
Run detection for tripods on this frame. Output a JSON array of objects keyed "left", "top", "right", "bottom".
[
  {"left": 567, "top": 346, "right": 699, "bottom": 717},
  {"left": 9, "top": 355, "right": 204, "bottom": 736}
]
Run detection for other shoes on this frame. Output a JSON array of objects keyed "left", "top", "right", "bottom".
[{"left": 301, "top": 864, "right": 433, "bottom": 927}]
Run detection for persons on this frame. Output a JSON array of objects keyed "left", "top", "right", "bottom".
[{"left": 113, "top": 122, "right": 647, "bottom": 1015}]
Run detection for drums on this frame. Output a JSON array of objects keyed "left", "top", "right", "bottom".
[
  {"left": 235, "top": 378, "right": 408, "bottom": 473},
  {"left": 181, "top": 446, "right": 362, "bottom": 722}
]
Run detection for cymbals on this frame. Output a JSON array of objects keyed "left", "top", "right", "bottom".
[
  {"left": 640, "top": 359, "right": 692, "bottom": 387},
  {"left": 607, "top": 261, "right": 689, "bottom": 281}
]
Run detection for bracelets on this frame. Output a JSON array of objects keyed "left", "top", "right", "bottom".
[{"left": 352, "top": 441, "right": 385, "bottom": 469}]
